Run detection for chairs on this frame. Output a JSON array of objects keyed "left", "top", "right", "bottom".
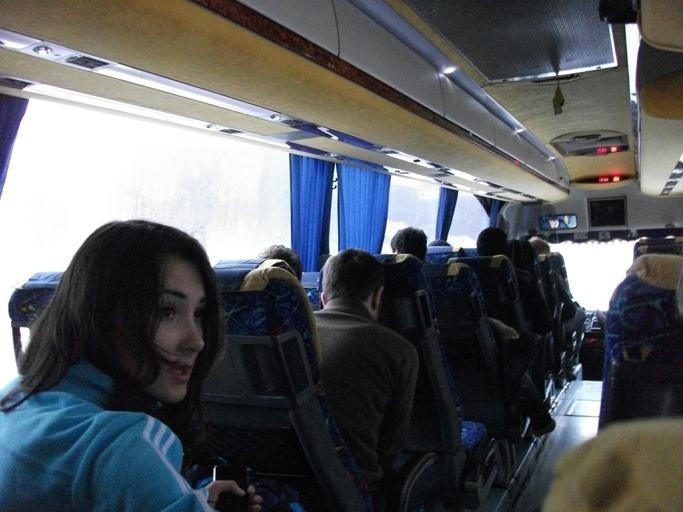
[{"left": 8, "top": 241, "right": 683, "bottom": 511}]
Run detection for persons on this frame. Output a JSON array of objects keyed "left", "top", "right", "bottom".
[
  {"left": 257, "top": 244, "right": 303, "bottom": 283},
  {"left": 542, "top": 215, "right": 566, "bottom": 228},
  {"left": 474, "top": 227, "right": 555, "bottom": 338},
  {"left": 0, "top": 217, "right": 267, "bottom": 512},
  {"left": 306, "top": 247, "right": 427, "bottom": 511},
  {"left": 389, "top": 226, "right": 557, "bottom": 440}
]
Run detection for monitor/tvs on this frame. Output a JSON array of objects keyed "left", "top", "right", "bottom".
[{"left": 587, "top": 197, "right": 627, "bottom": 231}]
